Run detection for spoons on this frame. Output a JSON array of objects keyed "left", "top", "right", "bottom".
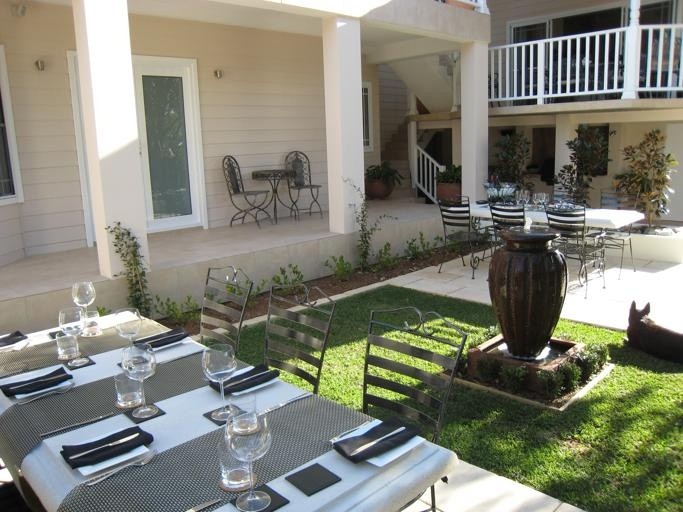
[{"left": 18, "top": 381, "right": 73, "bottom": 405}]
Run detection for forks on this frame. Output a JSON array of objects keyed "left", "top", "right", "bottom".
[{"left": 85, "top": 447, "right": 156, "bottom": 486}]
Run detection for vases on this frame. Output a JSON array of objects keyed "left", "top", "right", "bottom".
[{"left": 487, "top": 227, "right": 569, "bottom": 363}]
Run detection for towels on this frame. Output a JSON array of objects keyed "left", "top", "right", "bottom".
[
  {"left": 0, "top": 330, "right": 28, "bottom": 347},
  {"left": 133, "top": 327, "right": 189, "bottom": 348},
  {"left": 333, "top": 418, "right": 422, "bottom": 465},
  {"left": 207, "top": 363, "right": 279, "bottom": 396},
  {"left": 59, "top": 426, "right": 154, "bottom": 469},
  {"left": 0, "top": 366, "right": 73, "bottom": 397}
]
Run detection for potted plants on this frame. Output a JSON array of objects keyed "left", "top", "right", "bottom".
[
  {"left": 364, "top": 160, "right": 405, "bottom": 199},
  {"left": 435, "top": 164, "right": 462, "bottom": 204}
]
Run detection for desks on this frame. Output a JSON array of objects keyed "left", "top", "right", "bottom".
[
  {"left": 251, "top": 169, "right": 296, "bottom": 224},
  {"left": 0, "top": 309, "right": 458, "bottom": 512}
]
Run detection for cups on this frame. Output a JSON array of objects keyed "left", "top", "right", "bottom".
[
  {"left": 215, "top": 438, "right": 252, "bottom": 489},
  {"left": 229, "top": 393, "right": 258, "bottom": 432}
]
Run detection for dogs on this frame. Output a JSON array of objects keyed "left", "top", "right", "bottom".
[{"left": 625, "top": 300, "right": 683, "bottom": 363}]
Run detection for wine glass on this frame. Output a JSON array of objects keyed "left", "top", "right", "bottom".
[
  {"left": 486, "top": 188, "right": 575, "bottom": 213},
  {"left": 53, "top": 279, "right": 156, "bottom": 419},
  {"left": 226, "top": 409, "right": 272, "bottom": 511},
  {"left": 202, "top": 344, "right": 238, "bottom": 422}
]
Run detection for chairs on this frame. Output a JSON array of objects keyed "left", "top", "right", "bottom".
[
  {"left": 360, "top": 306, "right": 466, "bottom": 512},
  {"left": 222, "top": 155, "right": 274, "bottom": 228},
  {"left": 437, "top": 190, "right": 640, "bottom": 298},
  {"left": 284, "top": 151, "right": 323, "bottom": 221},
  {"left": 644, "top": 37, "right": 682, "bottom": 98},
  {"left": 200, "top": 265, "right": 253, "bottom": 359},
  {"left": 263, "top": 282, "right": 337, "bottom": 394}
]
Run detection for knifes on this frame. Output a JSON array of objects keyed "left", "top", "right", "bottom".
[
  {"left": 68, "top": 433, "right": 140, "bottom": 459},
  {"left": 184, "top": 499, "right": 222, "bottom": 511},
  {"left": 349, "top": 425, "right": 407, "bottom": 456},
  {"left": 144, "top": 333, "right": 182, "bottom": 345},
  {"left": 9, "top": 373, "right": 66, "bottom": 391},
  {"left": 223, "top": 369, "right": 271, "bottom": 390}
]
